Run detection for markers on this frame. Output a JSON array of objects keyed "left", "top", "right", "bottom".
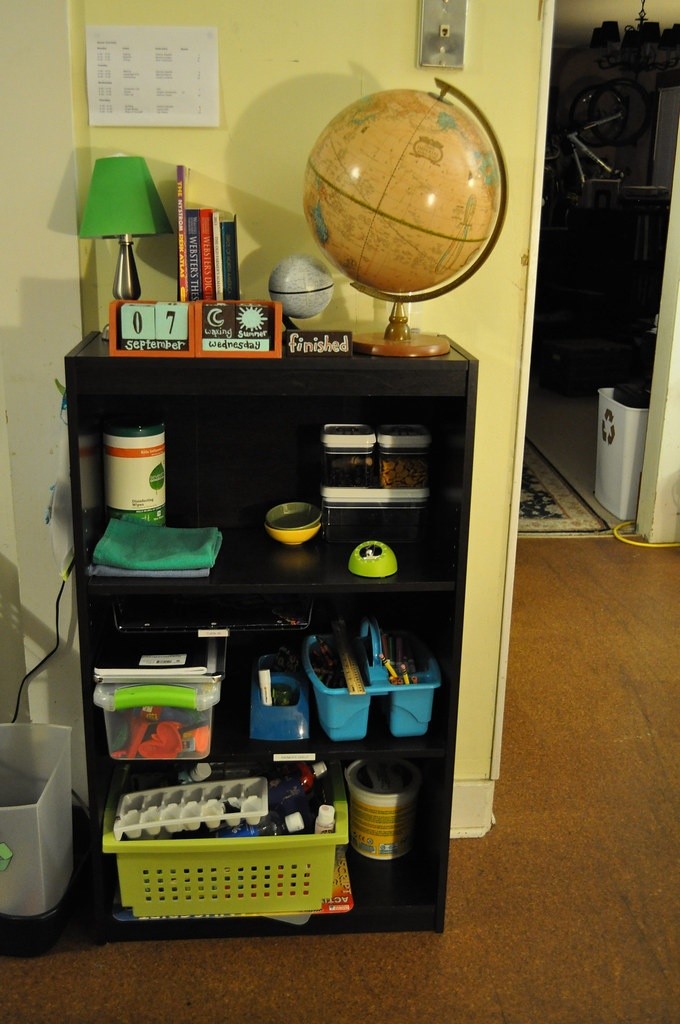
[{"left": 396, "top": 637, "right": 403, "bottom": 660}]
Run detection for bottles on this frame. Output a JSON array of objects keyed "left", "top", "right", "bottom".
[
  {"left": 215, "top": 810, "right": 304, "bottom": 838},
  {"left": 314, "top": 804, "right": 335, "bottom": 835},
  {"left": 254, "top": 761, "right": 327, "bottom": 808},
  {"left": 169, "top": 763, "right": 212, "bottom": 785}
]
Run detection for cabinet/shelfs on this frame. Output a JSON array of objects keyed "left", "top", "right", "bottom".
[{"left": 61, "top": 335, "right": 482, "bottom": 943}]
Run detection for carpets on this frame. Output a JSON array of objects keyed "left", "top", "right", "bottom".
[{"left": 517, "top": 433, "right": 620, "bottom": 541}]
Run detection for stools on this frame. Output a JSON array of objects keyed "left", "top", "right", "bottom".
[{"left": 539, "top": 339, "right": 633, "bottom": 399}]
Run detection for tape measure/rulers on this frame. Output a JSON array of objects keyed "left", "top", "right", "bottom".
[{"left": 331, "top": 620, "right": 367, "bottom": 695}]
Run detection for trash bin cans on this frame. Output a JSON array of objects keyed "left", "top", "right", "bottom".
[{"left": 594, "top": 383, "right": 651, "bottom": 521}]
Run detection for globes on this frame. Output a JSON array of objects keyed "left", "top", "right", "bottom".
[
  {"left": 268, "top": 256, "right": 334, "bottom": 346},
  {"left": 302, "top": 77, "right": 510, "bottom": 357}
]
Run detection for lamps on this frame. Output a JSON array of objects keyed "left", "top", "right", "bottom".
[
  {"left": 590, "top": 0, "right": 680, "bottom": 80},
  {"left": 79, "top": 156, "right": 179, "bottom": 341}
]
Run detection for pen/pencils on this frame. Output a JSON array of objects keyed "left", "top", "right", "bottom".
[
  {"left": 312, "top": 636, "right": 347, "bottom": 688},
  {"left": 388, "top": 634, "right": 395, "bottom": 659},
  {"left": 389, "top": 676, "right": 403, "bottom": 685},
  {"left": 401, "top": 664, "right": 410, "bottom": 684},
  {"left": 379, "top": 654, "right": 398, "bottom": 678},
  {"left": 411, "top": 676, "right": 418, "bottom": 683}
]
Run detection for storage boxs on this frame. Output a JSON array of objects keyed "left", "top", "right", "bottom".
[
  {"left": 0, "top": 723, "right": 74, "bottom": 918},
  {"left": 320, "top": 423, "right": 377, "bottom": 486},
  {"left": 89, "top": 681, "right": 222, "bottom": 763},
  {"left": 379, "top": 424, "right": 432, "bottom": 489},
  {"left": 320, "top": 482, "right": 431, "bottom": 543},
  {"left": 301, "top": 614, "right": 443, "bottom": 744},
  {"left": 100, "top": 758, "right": 352, "bottom": 916}
]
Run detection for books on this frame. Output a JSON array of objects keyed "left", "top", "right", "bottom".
[
  {"left": 625, "top": 209, "right": 666, "bottom": 310},
  {"left": 93, "top": 631, "right": 210, "bottom": 677},
  {"left": 176, "top": 164, "right": 242, "bottom": 302}
]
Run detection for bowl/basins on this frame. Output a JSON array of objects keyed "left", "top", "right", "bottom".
[{"left": 265, "top": 502, "right": 323, "bottom": 545}]
